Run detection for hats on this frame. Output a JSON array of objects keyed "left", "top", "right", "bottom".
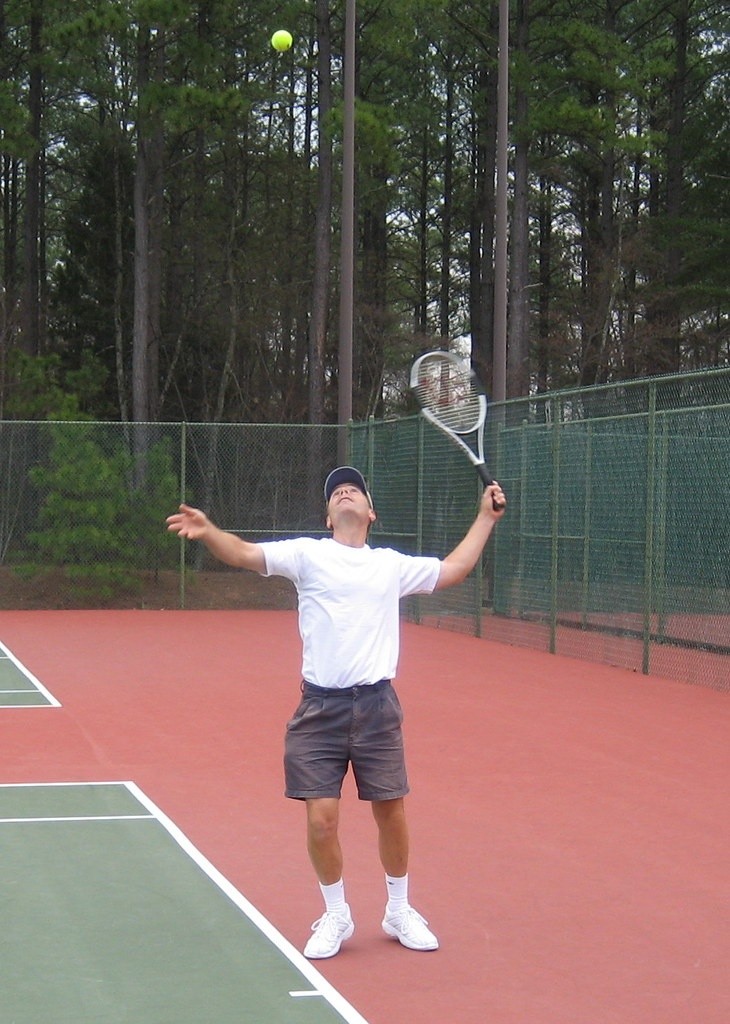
[{"left": 323, "top": 466, "right": 374, "bottom": 509}]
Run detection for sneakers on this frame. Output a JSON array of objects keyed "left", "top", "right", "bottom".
[
  {"left": 382, "top": 903, "right": 439, "bottom": 951},
  {"left": 304, "top": 903, "right": 354, "bottom": 959}
]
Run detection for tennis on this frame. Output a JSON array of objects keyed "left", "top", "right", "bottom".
[{"left": 271, "top": 29, "right": 294, "bottom": 52}]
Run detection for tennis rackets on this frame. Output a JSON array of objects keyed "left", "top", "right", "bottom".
[{"left": 411, "top": 350, "right": 505, "bottom": 512}]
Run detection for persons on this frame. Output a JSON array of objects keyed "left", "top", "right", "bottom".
[{"left": 165, "top": 466, "right": 506, "bottom": 960}]
